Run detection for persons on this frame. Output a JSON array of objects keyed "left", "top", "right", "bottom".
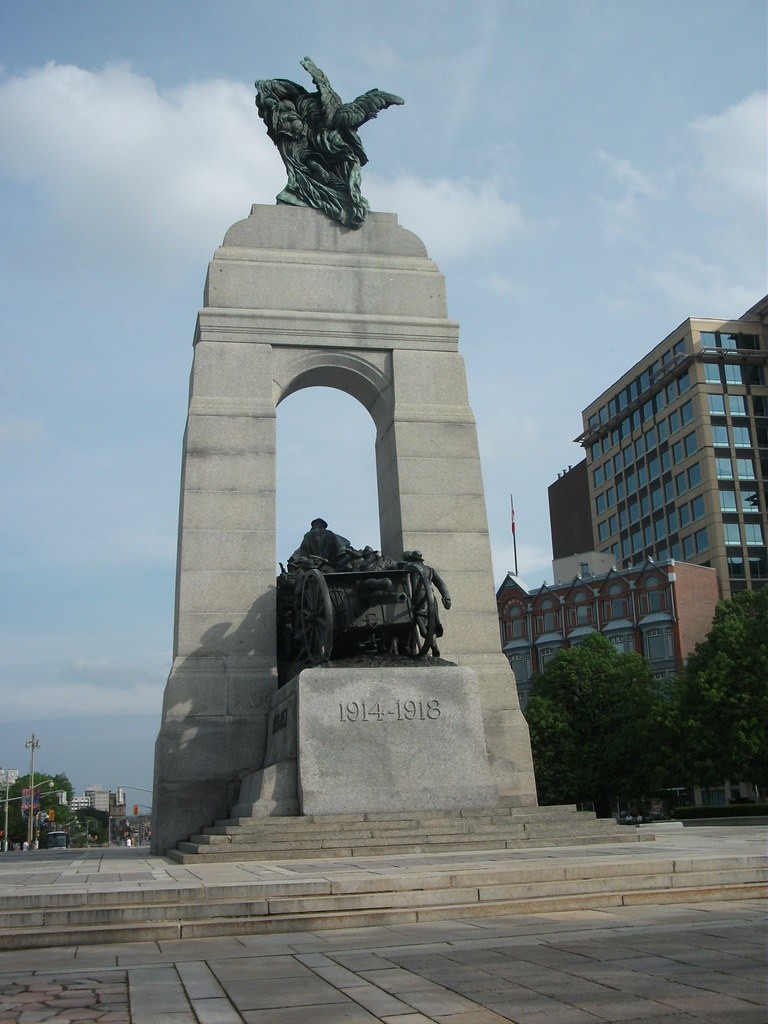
[
  {"left": 23, "top": 839, "right": 35, "bottom": 850},
  {"left": 288, "top": 519, "right": 452, "bottom": 656},
  {"left": 126, "top": 837, "right": 131, "bottom": 847},
  {"left": 65, "top": 832, "right": 71, "bottom": 848},
  {"left": 625, "top": 813, "right": 643, "bottom": 824}
]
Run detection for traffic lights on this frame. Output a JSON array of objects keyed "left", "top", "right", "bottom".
[
  {"left": 133, "top": 805, "right": 139, "bottom": 816},
  {"left": 49, "top": 810, "right": 54, "bottom": 820}
]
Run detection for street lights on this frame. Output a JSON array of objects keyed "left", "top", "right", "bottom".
[{"left": 24, "top": 732, "right": 54, "bottom": 849}]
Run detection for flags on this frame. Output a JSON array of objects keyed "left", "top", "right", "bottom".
[{"left": 510, "top": 502, "right": 516, "bottom": 533}]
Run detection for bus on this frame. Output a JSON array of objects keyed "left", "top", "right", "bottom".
[{"left": 45, "top": 831, "right": 70, "bottom": 850}]
